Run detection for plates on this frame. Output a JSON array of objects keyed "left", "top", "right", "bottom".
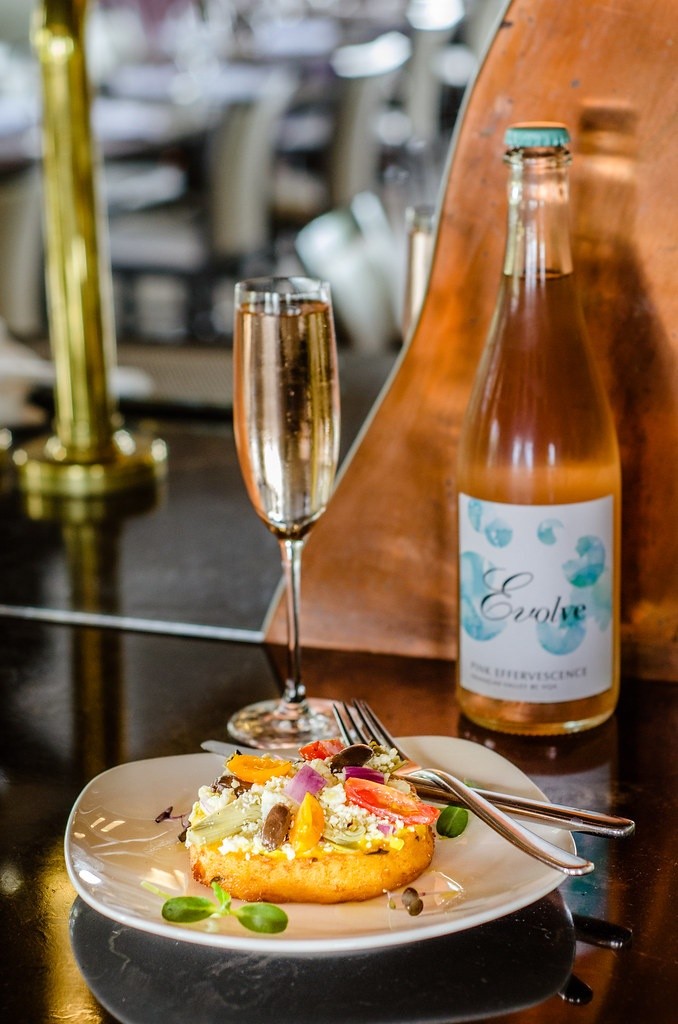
[{"left": 62, "top": 735, "right": 582, "bottom": 959}]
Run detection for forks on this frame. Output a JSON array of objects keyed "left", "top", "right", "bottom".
[{"left": 331, "top": 699, "right": 593, "bottom": 887}]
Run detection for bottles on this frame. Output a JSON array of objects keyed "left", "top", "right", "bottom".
[{"left": 453, "top": 123, "right": 623, "bottom": 735}]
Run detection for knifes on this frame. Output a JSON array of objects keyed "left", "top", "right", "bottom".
[{"left": 197, "top": 739, "right": 636, "bottom": 837}]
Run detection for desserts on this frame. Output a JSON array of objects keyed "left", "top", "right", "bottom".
[{"left": 181, "top": 735, "right": 440, "bottom": 903}]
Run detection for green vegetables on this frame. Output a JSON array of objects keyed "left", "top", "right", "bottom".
[
  {"left": 435, "top": 806, "right": 468, "bottom": 838},
  {"left": 138, "top": 879, "right": 288, "bottom": 934}
]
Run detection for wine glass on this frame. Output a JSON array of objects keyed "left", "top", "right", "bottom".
[{"left": 226, "top": 274, "right": 364, "bottom": 749}]
